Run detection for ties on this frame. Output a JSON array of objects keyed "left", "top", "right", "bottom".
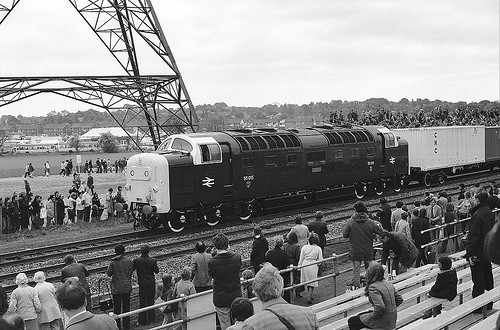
[{"left": 431, "top": 207, "right": 433, "bottom": 217}]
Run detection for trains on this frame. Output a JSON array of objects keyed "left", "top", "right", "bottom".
[{"left": 124, "top": 124, "right": 500, "bottom": 233}]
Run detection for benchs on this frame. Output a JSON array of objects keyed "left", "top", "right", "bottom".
[{"left": 304, "top": 249, "right": 499, "bottom": 330}]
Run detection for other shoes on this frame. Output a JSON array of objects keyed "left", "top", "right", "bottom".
[
  {"left": 23, "top": 153, "right": 130, "bottom": 180},
  {"left": 136, "top": 323, "right": 145, "bottom": 326},
  {"left": 294, "top": 297, "right": 302, "bottom": 300},
  {"left": 345, "top": 176, "right": 500, "bottom": 329},
  {"left": 308, "top": 300, "right": 313, "bottom": 303}
]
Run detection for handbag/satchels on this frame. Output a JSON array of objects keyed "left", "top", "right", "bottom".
[
  {"left": 99, "top": 280, "right": 113, "bottom": 310},
  {"left": 155, "top": 297, "right": 167, "bottom": 313}
]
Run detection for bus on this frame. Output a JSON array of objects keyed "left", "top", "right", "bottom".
[
  {"left": 16, "top": 143, "right": 57, "bottom": 154},
  {"left": 59, "top": 141, "right": 98, "bottom": 152}
]
